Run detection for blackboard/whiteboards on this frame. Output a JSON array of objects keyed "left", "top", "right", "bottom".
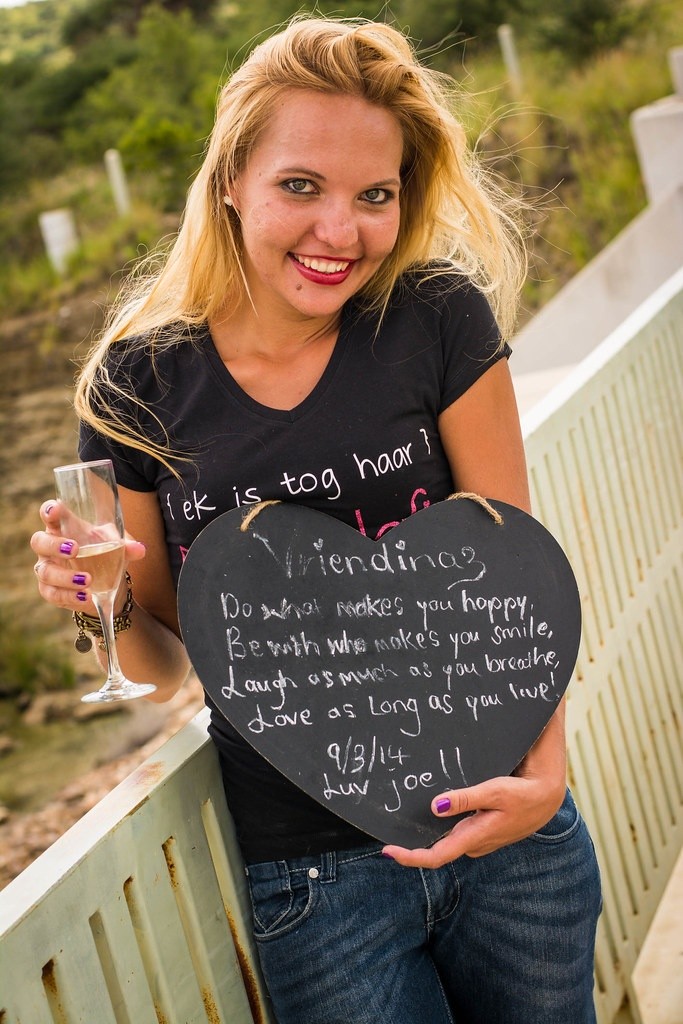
[{"left": 177, "top": 499, "right": 583, "bottom": 852}]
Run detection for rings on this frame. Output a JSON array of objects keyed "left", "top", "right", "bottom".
[{"left": 34, "top": 560, "right": 55, "bottom": 573}]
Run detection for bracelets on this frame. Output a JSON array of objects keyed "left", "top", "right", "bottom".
[{"left": 72, "top": 571, "right": 133, "bottom": 654}]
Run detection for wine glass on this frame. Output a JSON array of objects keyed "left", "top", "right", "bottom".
[{"left": 52, "top": 458, "right": 157, "bottom": 704}]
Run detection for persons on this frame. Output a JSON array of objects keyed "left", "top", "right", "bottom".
[{"left": 28, "top": 21, "right": 607, "bottom": 1024}]
